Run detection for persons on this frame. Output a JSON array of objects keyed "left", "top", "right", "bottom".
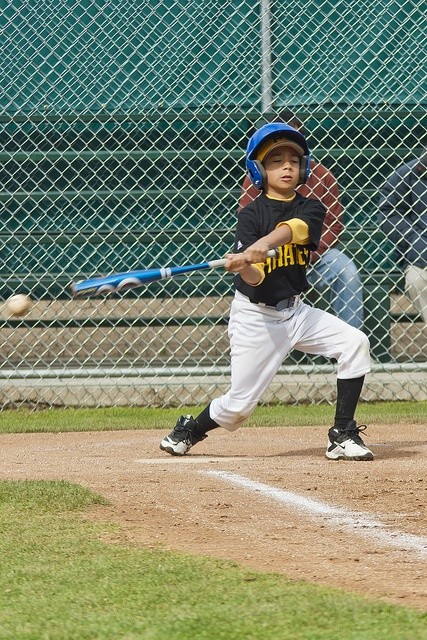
[
  {"left": 159, "top": 122, "right": 375, "bottom": 461},
  {"left": 236, "top": 110, "right": 365, "bottom": 332},
  {"left": 372, "top": 146, "right": 427, "bottom": 322}
]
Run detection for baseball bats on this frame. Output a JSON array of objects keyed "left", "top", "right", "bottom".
[{"left": 73, "top": 249, "right": 277, "bottom": 299}]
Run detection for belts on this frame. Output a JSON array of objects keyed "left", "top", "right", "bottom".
[{"left": 250, "top": 298, "right": 295, "bottom": 311}]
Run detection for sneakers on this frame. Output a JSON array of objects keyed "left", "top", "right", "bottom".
[
  {"left": 161, "top": 414, "right": 207, "bottom": 454},
  {"left": 325, "top": 421, "right": 377, "bottom": 462}
]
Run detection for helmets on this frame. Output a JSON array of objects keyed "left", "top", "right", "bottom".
[{"left": 246, "top": 122, "right": 310, "bottom": 188}]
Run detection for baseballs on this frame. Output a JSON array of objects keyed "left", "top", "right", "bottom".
[{"left": 9, "top": 294, "right": 32, "bottom": 316}]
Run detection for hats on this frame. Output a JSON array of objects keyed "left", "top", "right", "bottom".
[{"left": 256, "top": 136, "right": 304, "bottom": 164}]
